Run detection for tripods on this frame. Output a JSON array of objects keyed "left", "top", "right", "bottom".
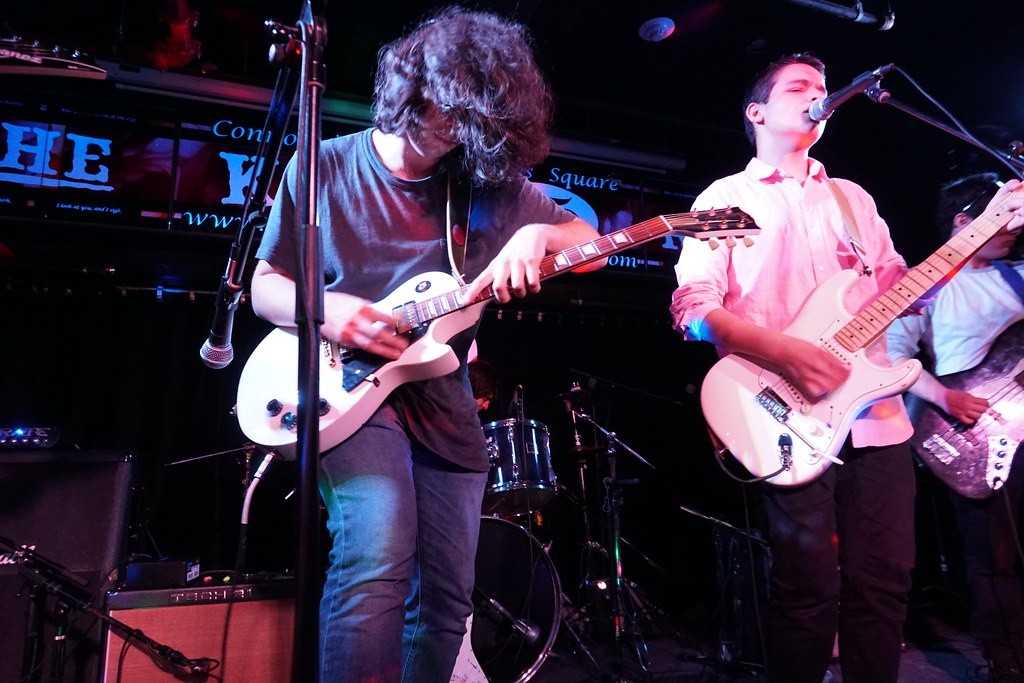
[{"left": 559, "top": 399, "right": 771, "bottom": 683}]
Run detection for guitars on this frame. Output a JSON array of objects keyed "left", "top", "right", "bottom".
[
  {"left": 911, "top": 342, "right": 1024, "bottom": 499},
  {"left": 235, "top": 203, "right": 760, "bottom": 462},
  {"left": 698, "top": 180, "right": 1024, "bottom": 488}
]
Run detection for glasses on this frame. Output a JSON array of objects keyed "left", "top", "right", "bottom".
[{"left": 961, "top": 181, "right": 1005, "bottom": 212}]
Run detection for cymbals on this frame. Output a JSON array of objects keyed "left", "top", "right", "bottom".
[{"left": 553, "top": 445, "right": 607, "bottom": 469}]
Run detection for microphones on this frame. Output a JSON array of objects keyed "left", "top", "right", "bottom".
[
  {"left": 514, "top": 618, "right": 541, "bottom": 644},
  {"left": 191, "top": 664, "right": 210, "bottom": 677},
  {"left": 809, "top": 63, "right": 894, "bottom": 121},
  {"left": 200, "top": 242, "right": 240, "bottom": 369},
  {"left": 508, "top": 384, "right": 522, "bottom": 415}
]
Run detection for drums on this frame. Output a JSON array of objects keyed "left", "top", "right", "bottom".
[
  {"left": 481, "top": 419, "right": 559, "bottom": 510},
  {"left": 469, "top": 517, "right": 562, "bottom": 683}
]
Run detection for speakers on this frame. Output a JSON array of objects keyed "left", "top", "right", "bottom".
[
  {"left": 101, "top": 577, "right": 308, "bottom": 683},
  {"left": 0, "top": 450, "right": 137, "bottom": 683},
  {"left": 712, "top": 521, "right": 840, "bottom": 668}
]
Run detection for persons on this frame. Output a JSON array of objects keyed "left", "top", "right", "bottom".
[
  {"left": 671, "top": 52, "right": 1024, "bottom": 683},
  {"left": 881, "top": 172, "right": 1024, "bottom": 683},
  {"left": 248, "top": 16, "right": 608, "bottom": 683}
]
